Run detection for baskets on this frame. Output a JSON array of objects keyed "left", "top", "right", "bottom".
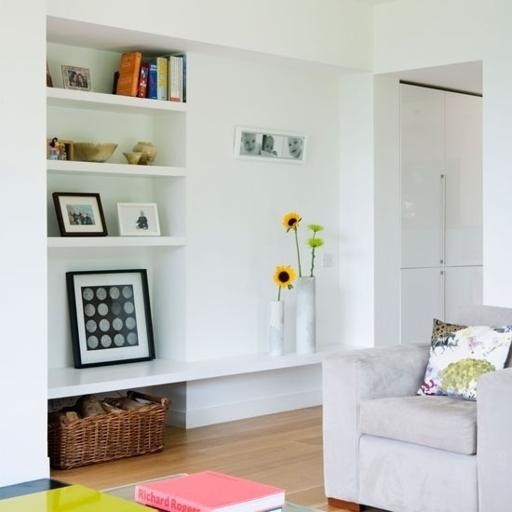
[{"left": 48, "top": 392, "right": 171, "bottom": 470}]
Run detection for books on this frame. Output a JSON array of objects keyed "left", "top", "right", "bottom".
[
  {"left": 115, "top": 49, "right": 185, "bottom": 103},
  {"left": 133, "top": 469, "right": 285, "bottom": 512}
]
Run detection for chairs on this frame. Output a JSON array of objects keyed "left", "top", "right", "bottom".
[{"left": 320, "top": 303, "right": 512, "bottom": 512}]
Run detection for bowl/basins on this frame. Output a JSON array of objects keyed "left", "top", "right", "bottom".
[
  {"left": 123, "top": 152, "right": 143, "bottom": 164},
  {"left": 74, "top": 141, "right": 120, "bottom": 163}
]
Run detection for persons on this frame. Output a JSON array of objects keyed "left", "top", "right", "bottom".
[
  {"left": 78, "top": 211, "right": 85, "bottom": 225},
  {"left": 287, "top": 137, "right": 303, "bottom": 159},
  {"left": 259, "top": 135, "right": 279, "bottom": 157},
  {"left": 77, "top": 74, "right": 87, "bottom": 87},
  {"left": 69, "top": 70, "right": 78, "bottom": 86},
  {"left": 69, "top": 209, "right": 79, "bottom": 224},
  {"left": 136, "top": 211, "right": 148, "bottom": 230},
  {"left": 241, "top": 131, "right": 259, "bottom": 155},
  {"left": 85, "top": 213, "right": 93, "bottom": 224}
]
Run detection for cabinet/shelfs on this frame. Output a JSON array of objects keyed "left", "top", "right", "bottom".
[
  {"left": 48, "top": 78, "right": 187, "bottom": 246},
  {"left": 400, "top": 81, "right": 485, "bottom": 344}
]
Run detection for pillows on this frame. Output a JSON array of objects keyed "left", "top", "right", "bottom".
[{"left": 415, "top": 316, "right": 511, "bottom": 402}]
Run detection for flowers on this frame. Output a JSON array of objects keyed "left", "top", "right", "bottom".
[{"left": 272, "top": 212, "right": 326, "bottom": 301}]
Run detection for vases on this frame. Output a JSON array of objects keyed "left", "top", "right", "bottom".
[{"left": 269, "top": 275, "right": 318, "bottom": 358}]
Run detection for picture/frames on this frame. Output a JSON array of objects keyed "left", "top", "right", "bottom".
[
  {"left": 52, "top": 192, "right": 161, "bottom": 238},
  {"left": 65, "top": 269, "right": 156, "bottom": 369},
  {"left": 234, "top": 126, "right": 308, "bottom": 164}
]
